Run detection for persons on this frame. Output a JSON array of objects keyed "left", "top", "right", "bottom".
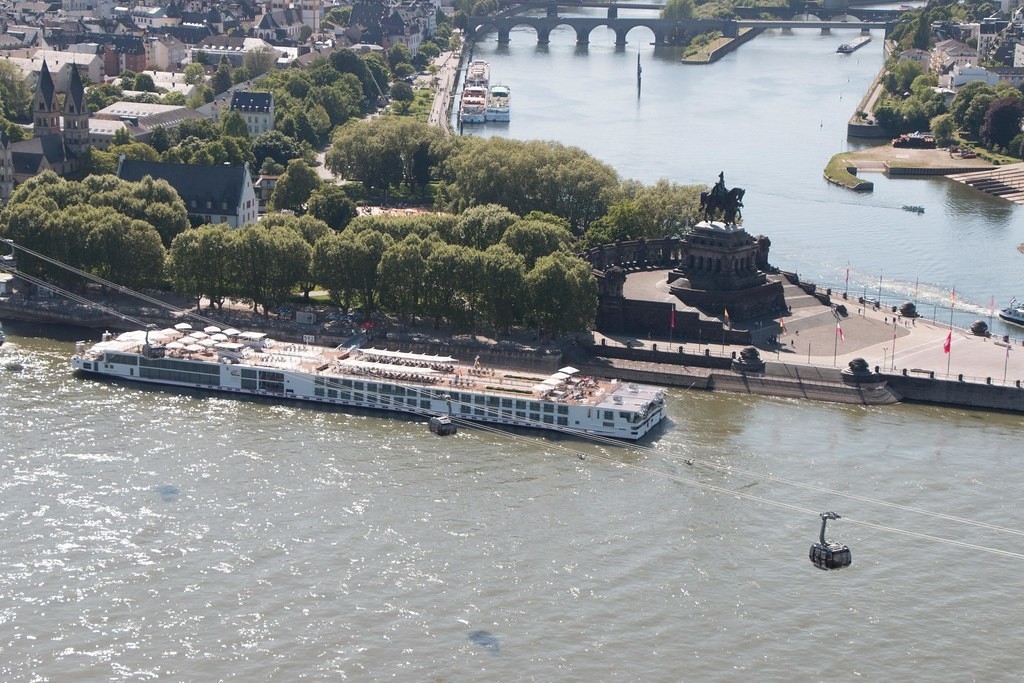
[
  {"left": 707, "top": 172, "right": 728, "bottom": 213},
  {"left": 771, "top": 327, "right": 798, "bottom": 347}
]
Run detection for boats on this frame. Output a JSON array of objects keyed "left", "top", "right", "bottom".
[
  {"left": 465, "top": 60, "right": 491, "bottom": 85},
  {"left": 486, "top": 84, "right": 511, "bottom": 122},
  {"left": 68, "top": 321, "right": 671, "bottom": 440},
  {"left": 836, "top": 35, "right": 873, "bottom": 52},
  {"left": 459, "top": 85, "right": 488, "bottom": 124},
  {"left": 998, "top": 295, "right": 1024, "bottom": 330}
]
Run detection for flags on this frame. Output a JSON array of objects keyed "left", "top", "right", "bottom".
[
  {"left": 780, "top": 317, "right": 787, "bottom": 336},
  {"left": 722, "top": 309, "right": 730, "bottom": 330},
  {"left": 944, "top": 333, "right": 951, "bottom": 353},
  {"left": 950, "top": 288, "right": 955, "bottom": 307},
  {"left": 991, "top": 298, "right": 995, "bottom": 314},
  {"left": 837, "top": 323, "right": 844, "bottom": 341}
]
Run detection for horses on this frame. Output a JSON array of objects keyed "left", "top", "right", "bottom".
[{"left": 697, "top": 187, "right": 746, "bottom": 224}]
